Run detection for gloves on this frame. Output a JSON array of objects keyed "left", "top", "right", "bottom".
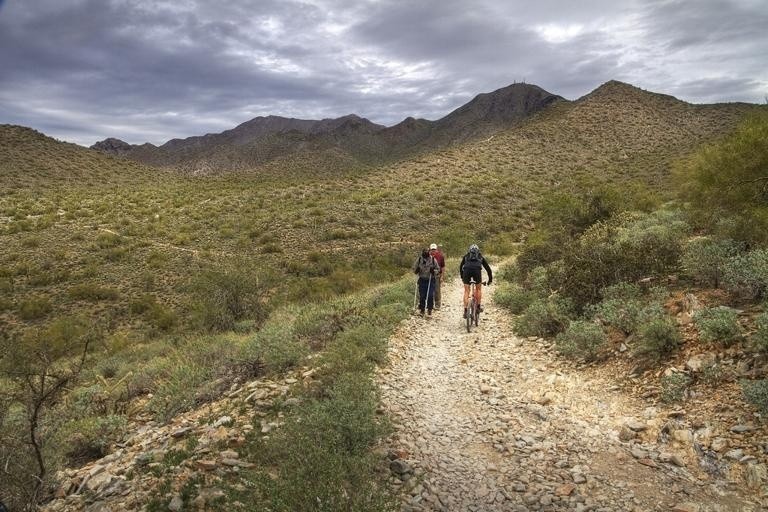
[
  {"left": 416, "top": 267, "right": 420, "bottom": 273},
  {"left": 430, "top": 267, "right": 434, "bottom": 273}
]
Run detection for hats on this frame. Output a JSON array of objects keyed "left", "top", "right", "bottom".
[{"left": 430, "top": 244, "right": 437, "bottom": 249}]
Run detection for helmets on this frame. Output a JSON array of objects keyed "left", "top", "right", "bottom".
[{"left": 469, "top": 244, "right": 479, "bottom": 251}]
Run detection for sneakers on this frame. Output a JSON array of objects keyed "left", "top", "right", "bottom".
[
  {"left": 463, "top": 314, "right": 467, "bottom": 319},
  {"left": 477, "top": 309, "right": 483, "bottom": 313},
  {"left": 418, "top": 303, "right": 441, "bottom": 314}
]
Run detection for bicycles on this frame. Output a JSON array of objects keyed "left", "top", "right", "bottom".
[{"left": 462, "top": 281, "right": 488, "bottom": 332}]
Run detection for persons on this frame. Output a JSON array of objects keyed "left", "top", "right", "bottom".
[
  {"left": 413, "top": 248, "right": 440, "bottom": 315},
  {"left": 427, "top": 243, "right": 446, "bottom": 311},
  {"left": 458, "top": 244, "right": 492, "bottom": 320}
]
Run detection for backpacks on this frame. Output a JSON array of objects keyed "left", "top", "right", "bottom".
[{"left": 468, "top": 248, "right": 480, "bottom": 260}]
